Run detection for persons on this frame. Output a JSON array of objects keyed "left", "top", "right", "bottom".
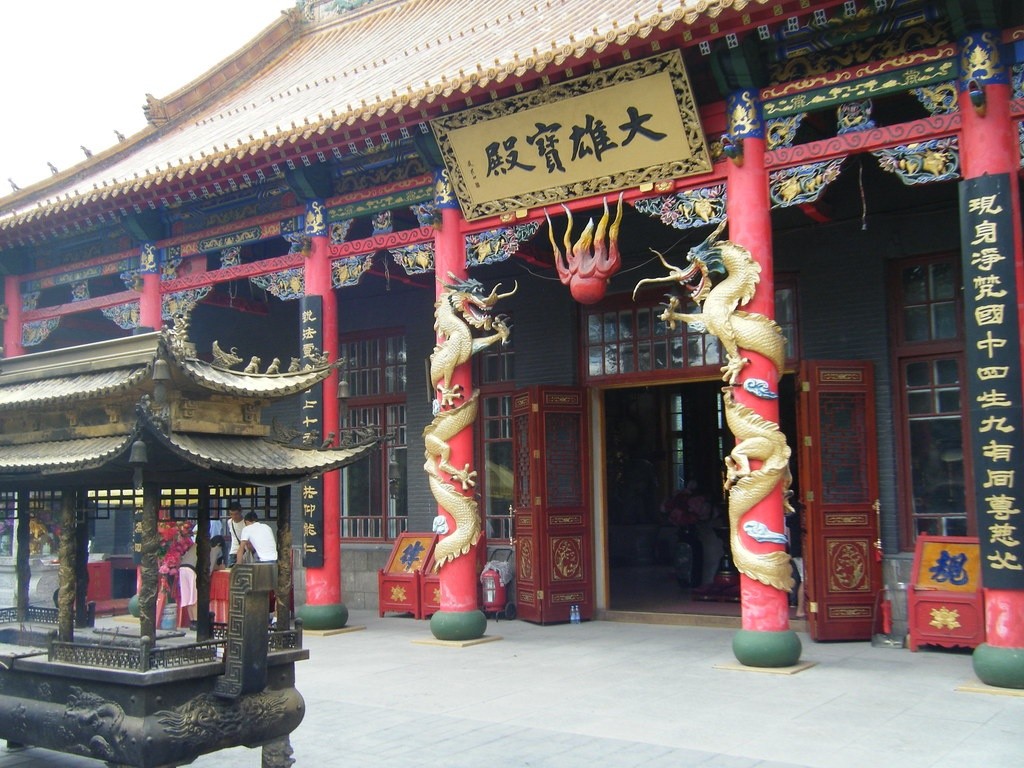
[
  {"left": 227, "top": 502, "right": 246, "bottom": 568},
  {"left": 235, "top": 512, "right": 278, "bottom": 624},
  {"left": 210, "top": 515, "right": 222, "bottom": 540},
  {"left": 179, "top": 535, "right": 224, "bottom": 632}
]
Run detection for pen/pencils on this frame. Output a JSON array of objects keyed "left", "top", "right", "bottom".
[{"left": 222, "top": 559, "right": 223, "bottom": 564}]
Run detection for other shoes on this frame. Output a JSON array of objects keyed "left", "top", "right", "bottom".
[{"left": 190, "top": 624, "right": 198, "bottom": 631}]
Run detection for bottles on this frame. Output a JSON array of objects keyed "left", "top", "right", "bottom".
[
  {"left": 570, "top": 606, "right": 575, "bottom": 624},
  {"left": 575, "top": 605, "right": 580, "bottom": 624}
]
